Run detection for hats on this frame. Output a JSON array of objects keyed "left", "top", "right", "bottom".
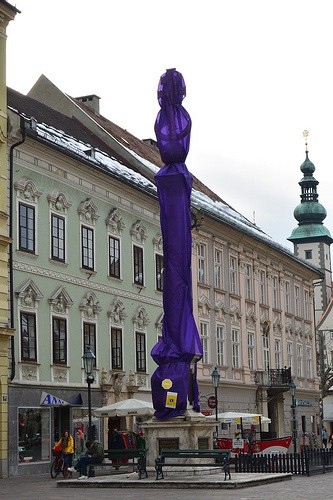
[{"left": 88, "top": 438, "right": 95, "bottom": 445}]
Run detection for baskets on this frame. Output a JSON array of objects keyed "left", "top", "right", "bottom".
[{"left": 52, "top": 449, "right": 63, "bottom": 456}]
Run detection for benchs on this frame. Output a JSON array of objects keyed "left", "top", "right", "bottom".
[
  {"left": 155, "top": 447, "right": 232, "bottom": 481},
  {"left": 88, "top": 449, "right": 149, "bottom": 479}
]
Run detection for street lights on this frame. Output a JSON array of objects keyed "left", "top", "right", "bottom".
[
  {"left": 211, "top": 366, "right": 221, "bottom": 441},
  {"left": 79, "top": 345, "right": 95, "bottom": 427},
  {"left": 289, "top": 382, "right": 297, "bottom": 474}
]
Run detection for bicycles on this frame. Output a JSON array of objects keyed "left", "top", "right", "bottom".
[{"left": 50, "top": 448, "right": 71, "bottom": 479}]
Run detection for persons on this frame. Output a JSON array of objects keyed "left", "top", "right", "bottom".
[
  {"left": 322, "top": 428, "right": 328, "bottom": 448},
  {"left": 137, "top": 438, "right": 149, "bottom": 479},
  {"left": 53, "top": 430, "right": 74, "bottom": 478},
  {"left": 303, "top": 432, "right": 310, "bottom": 452},
  {"left": 67, "top": 442, "right": 104, "bottom": 479}
]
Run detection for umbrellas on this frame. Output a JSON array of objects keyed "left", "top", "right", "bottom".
[{"left": 94, "top": 399, "right": 155, "bottom": 432}]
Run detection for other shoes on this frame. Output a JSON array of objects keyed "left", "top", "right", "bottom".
[
  {"left": 77, "top": 475, "right": 88, "bottom": 479},
  {"left": 67, "top": 466, "right": 77, "bottom": 472}
]
